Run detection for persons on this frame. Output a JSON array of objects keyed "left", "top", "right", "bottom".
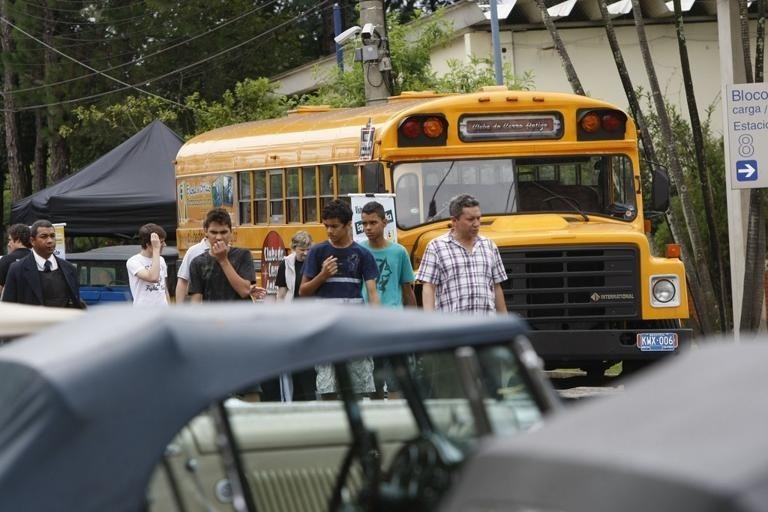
[
  {"left": 356, "top": 200, "right": 418, "bottom": 400},
  {"left": 0, "top": 224, "right": 32, "bottom": 300},
  {"left": 174, "top": 220, "right": 265, "bottom": 402},
  {"left": 2, "top": 219, "right": 82, "bottom": 307},
  {"left": 274, "top": 230, "right": 314, "bottom": 401},
  {"left": 299, "top": 199, "right": 380, "bottom": 400},
  {"left": 419, "top": 192, "right": 511, "bottom": 401},
  {"left": 187, "top": 208, "right": 257, "bottom": 300},
  {"left": 126, "top": 224, "right": 173, "bottom": 304}
]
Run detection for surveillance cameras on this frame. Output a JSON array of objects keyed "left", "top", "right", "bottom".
[
  {"left": 334, "top": 26, "right": 360, "bottom": 48},
  {"left": 362, "top": 23, "right": 374, "bottom": 41}
]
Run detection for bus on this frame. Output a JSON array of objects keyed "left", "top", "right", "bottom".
[{"left": 174, "top": 85, "right": 701, "bottom": 379}]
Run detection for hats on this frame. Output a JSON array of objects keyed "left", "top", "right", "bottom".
[{"left": 291, "top": 231, "right": 312, "bottom": 248}]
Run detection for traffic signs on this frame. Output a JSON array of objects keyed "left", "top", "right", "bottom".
[{"left": 725, "top": 82, "right": 768, "bottom": 191}]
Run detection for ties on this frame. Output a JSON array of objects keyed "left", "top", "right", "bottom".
[{"left": 44, "top": 260, "right": 52, "bottom": 272}]
